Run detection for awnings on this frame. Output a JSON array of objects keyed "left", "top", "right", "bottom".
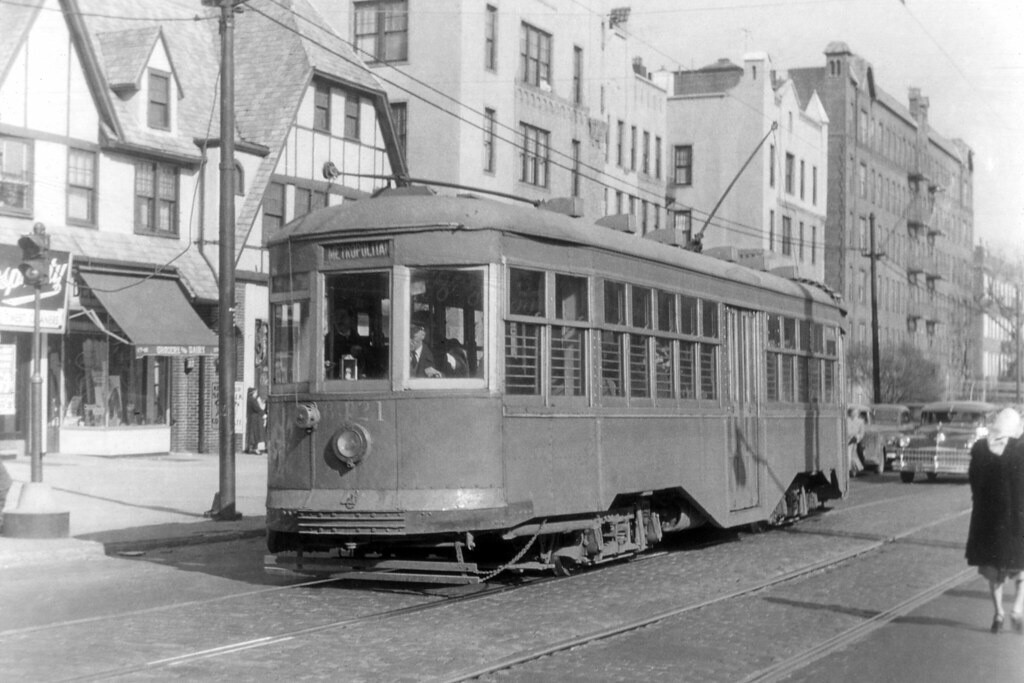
[{"left": 79, "top": 265, "right": 219, "bottom": 359}]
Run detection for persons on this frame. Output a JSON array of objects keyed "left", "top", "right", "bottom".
[
  {"left": 246, "top": 387, "right": 266, "bottom": 455},
  {"left": 845, "top": 407, "right": 865, "bottom": 477},
  {"left": 385, "top": 318, "right": 447, "bottom": 379},
  {"left": 324, "top": 303, "right": 381, "bottom": 380},
  {"left": 963, "top": 407, "right": 1024, "bottom": 634}
]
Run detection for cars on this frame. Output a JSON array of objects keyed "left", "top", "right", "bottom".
[
  {"left": 892, "top": 399, "right": 997, "bottom": 482},
  {"left": 855, "top": 403, "right": 920, "bottom": 474}
]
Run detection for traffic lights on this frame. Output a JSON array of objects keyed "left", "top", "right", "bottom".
[{"left": 15, "top": 234, "right": 49, "bottom": 285}]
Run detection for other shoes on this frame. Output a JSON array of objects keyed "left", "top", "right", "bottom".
[{"left": 252, "top": 449, "right": 262, "bottom": 455}]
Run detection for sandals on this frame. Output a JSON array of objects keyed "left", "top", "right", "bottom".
[
  {"left": 991, "top": 612, "right": 1004, "bottom": 634},
  {"left": 1010, "top": 610, "right": 1023, "bottom": 632}
]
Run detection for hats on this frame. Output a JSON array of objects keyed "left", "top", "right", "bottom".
[{"left": 411, "top": 319, "right": 426, "bottom": 331}]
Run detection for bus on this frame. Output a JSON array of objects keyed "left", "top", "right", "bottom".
[{"left": 262, "top": 187, "right": 849, "bottom": 597}]
